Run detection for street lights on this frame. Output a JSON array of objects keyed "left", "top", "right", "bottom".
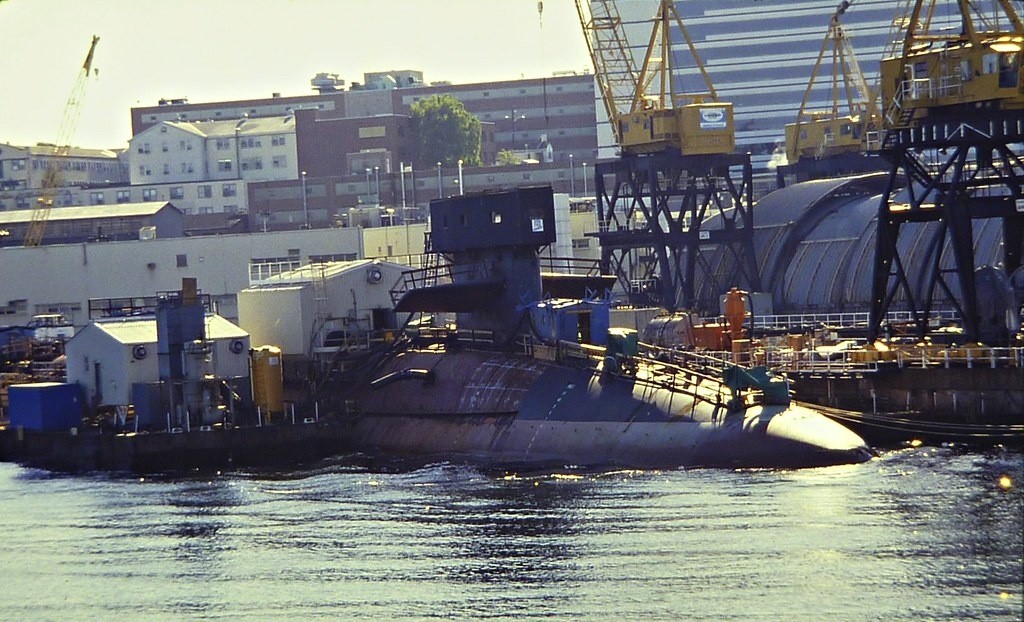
[
  {"left": 457, "top": 161, "right": 463, "bottom": 196},
  {"left": 301, "top": 171, "right": 308, "bottom": 227}
]
